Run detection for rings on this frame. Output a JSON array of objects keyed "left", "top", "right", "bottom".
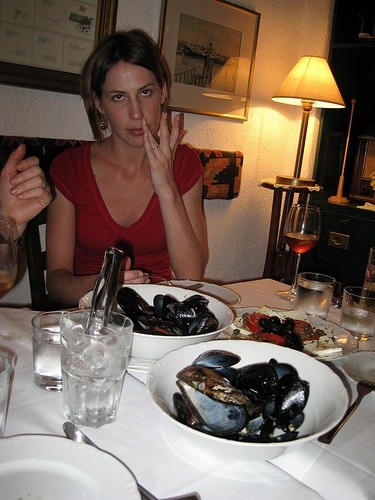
[
  {"left": 153, "top": 145, "right": 159, "bottom": 150},
  {"left": 44, "top": 180, "right": 47, "bottom": 189}
]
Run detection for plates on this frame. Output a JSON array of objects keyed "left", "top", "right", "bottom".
[
  {"left": 340, "top": 351, "right": 375, "bottom": 384},
  {"left": 235, "top": 307, "right": 357, "bottom": 362},
  {"left": 154, "top": 280, "right": 241, "bottom": 308},
  {"left": 0, "top": 434, "right": 142, "bottom": 499}
]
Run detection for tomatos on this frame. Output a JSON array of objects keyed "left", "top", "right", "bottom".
[{"left": 246, "top": 311, "right": 320, "bottom": 346}]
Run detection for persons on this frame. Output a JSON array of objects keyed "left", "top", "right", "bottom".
[
  {"left": 0, "top": 143, "right": 52, "bottom": 270},
  {"left": 46, "top": 29, "right": 209, "bottom": 305}
]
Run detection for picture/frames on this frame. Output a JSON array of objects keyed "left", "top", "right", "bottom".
[
  {"left": 0, "top": 0, "right": 118, "bottom": 94},
  {"left": 157, "top": 0, "right": 261, "bottom": 122}
]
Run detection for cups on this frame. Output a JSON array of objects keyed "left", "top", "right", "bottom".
[
  {"left": 31, "top": 311, "right": 70, "bottom": 392},
  {"left": 0, "top": 352, "right": 12, "bottom": 438},
  {"left": 59, "top": 308, "right": 134, "bottom": 428},
  {"left": 339, "top": 286, "right": 375, "bottom": 340},
  {"left": 294, "top": 272, "right": 336, "bottom": 320}
]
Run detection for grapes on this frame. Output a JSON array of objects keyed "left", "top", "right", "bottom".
[{"left": 258, "top": 316, "right": 294, "bottom": 335}]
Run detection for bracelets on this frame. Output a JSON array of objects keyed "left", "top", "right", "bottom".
[{"left": 0, "top": 234, "right": 16, "bottom": 244}]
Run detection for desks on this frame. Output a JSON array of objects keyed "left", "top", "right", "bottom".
[{"left": 258, "top": 180, "right": 323, "bottom": 280}]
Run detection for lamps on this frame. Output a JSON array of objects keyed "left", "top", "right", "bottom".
[
  {"left": 202, "top": 56, "right": 251, "bottom": 102},
  {"left": 271, "top": 53, "right": 348, "bottom": 186}
]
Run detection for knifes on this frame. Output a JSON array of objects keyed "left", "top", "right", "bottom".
[{"left": 64, "top": 421, "right": 157, "bottom": 500}]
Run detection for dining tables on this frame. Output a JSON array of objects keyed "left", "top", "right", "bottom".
[{"left": 0, "top": 277, "right": 375, "bottom": 500}]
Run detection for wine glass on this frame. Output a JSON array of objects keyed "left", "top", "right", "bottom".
[
  {"left": 274, "top": 204, "right": 321, "bottom": 301},
  {"left": 0, "top": 219, "right": 18, "bottom": 364}
]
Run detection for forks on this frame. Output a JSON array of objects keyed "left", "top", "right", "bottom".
[{"left": 317, "top": 380, "right": 375, "bottom": 445}]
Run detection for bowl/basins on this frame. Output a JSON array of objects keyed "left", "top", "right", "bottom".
[
  {"left": 77, "top": 283, "right": 234, "bottom": 361},
  {"left": 145, "top": 339, "right": 350, "bottom": 461}
]
